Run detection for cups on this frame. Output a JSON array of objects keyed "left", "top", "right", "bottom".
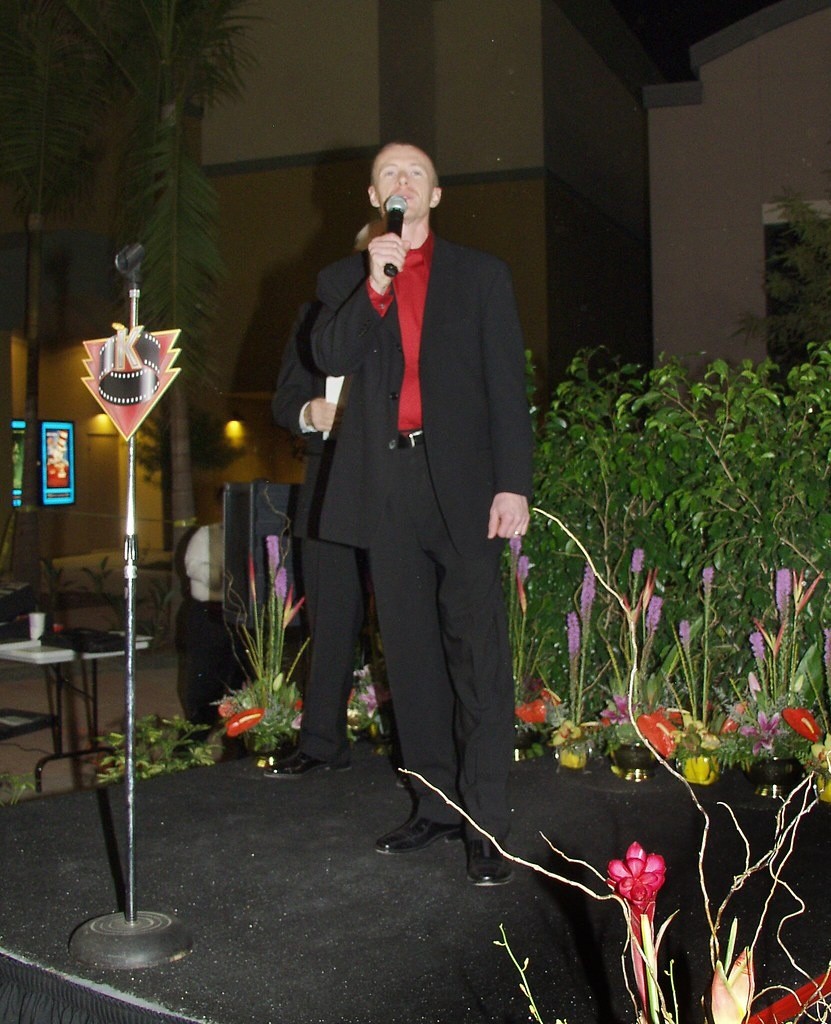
[{"left": 28, "top": 612, "right": 46, "bottom": 640}]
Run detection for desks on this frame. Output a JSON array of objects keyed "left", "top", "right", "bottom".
[{"left": 0, "top": 630, "right": 154, "bottom": 794}]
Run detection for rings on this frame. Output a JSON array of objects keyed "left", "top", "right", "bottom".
[{"left": 515, "top": 530, "right": 521, "bottom": 536}]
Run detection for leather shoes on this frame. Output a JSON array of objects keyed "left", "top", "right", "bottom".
[
  {"left": 264, "top": 748, "right": 352, "bottom": 780},
  {"left": 375, "top": 815, "right": 463, "bottom": 854},
  {"left": 467, "top": 838, "right": 515, "bottom": 888}
]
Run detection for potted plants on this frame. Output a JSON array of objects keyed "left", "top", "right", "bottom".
[{"left": 81, "top": 547, "right": 180, "bottom": 672}]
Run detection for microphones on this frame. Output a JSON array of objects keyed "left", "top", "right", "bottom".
[{"left": 384, "top": 196, "right": 408, "bottom": 277}]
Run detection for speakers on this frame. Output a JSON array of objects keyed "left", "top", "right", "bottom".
[{"left": 222, "top": 481, "right": 304, "bottom": 628}]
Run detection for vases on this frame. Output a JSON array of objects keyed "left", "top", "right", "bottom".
[
  {"left": 680, "top": 754, "right": 719, "bottom": 786},
  {"left": 506, "top": 724, "right": 544, "bottom": 761},
  {"left": 609, "top": 741, "right": 662, "bottom": 784},
  {"left": 341, "top": 723, "right": 377, "bottom": 742},
  {"left": 255, "top": 751, "right": 284, "bottom": 770},
  {"left": 752, "top": 757, "right": 798, "bottom": 798},
  {"left": 550, "top": 721, "right": 596, "bottom": 770},
  {"left": 814, "top": 771, "right": 831, "bottom": 803}
]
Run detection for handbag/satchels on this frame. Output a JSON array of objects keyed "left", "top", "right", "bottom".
[{"left": 37, "top": 626, "right": 125, "bottom": 653}]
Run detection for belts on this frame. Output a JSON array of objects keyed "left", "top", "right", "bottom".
[{"left": 398, "top": 430, "right": 425, "bottom": 448}]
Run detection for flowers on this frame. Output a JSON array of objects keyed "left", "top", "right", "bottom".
[
  {"left": 659, "top": 566, "right": 726, "bottom": 781},
  {"left": 395, "top": 508, "right": 831, "bottom": 1024},
  {"left": 601, "top": 544, "right": 717, "bottom": 756},
  {"left": 347, "top": 595, "right": 391, "bottom": 736},
  {"left": 780, "top": 628, "right": 831, "bottom": 785},
  {"left": 711, "top": 567, "right": 825, "bottom": 774},
  {"left": 210, "top": 481, "right": 305, "bottom": 751},
  {"left": 548, "top": 560, "right": 597, "bottom": 758},
  {"left": 500, "top": 533, "right": 534, "bottom": 724}
]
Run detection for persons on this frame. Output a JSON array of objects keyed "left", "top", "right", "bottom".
[
  {"left": 309, "top": 137, "right": 534, "bottom": 885},
  {"left": 265, "top": 220, "right": 458, "bottom": 787},
  {"left": 174, "top": 489, "right": 229, "bottom": 753}
]
